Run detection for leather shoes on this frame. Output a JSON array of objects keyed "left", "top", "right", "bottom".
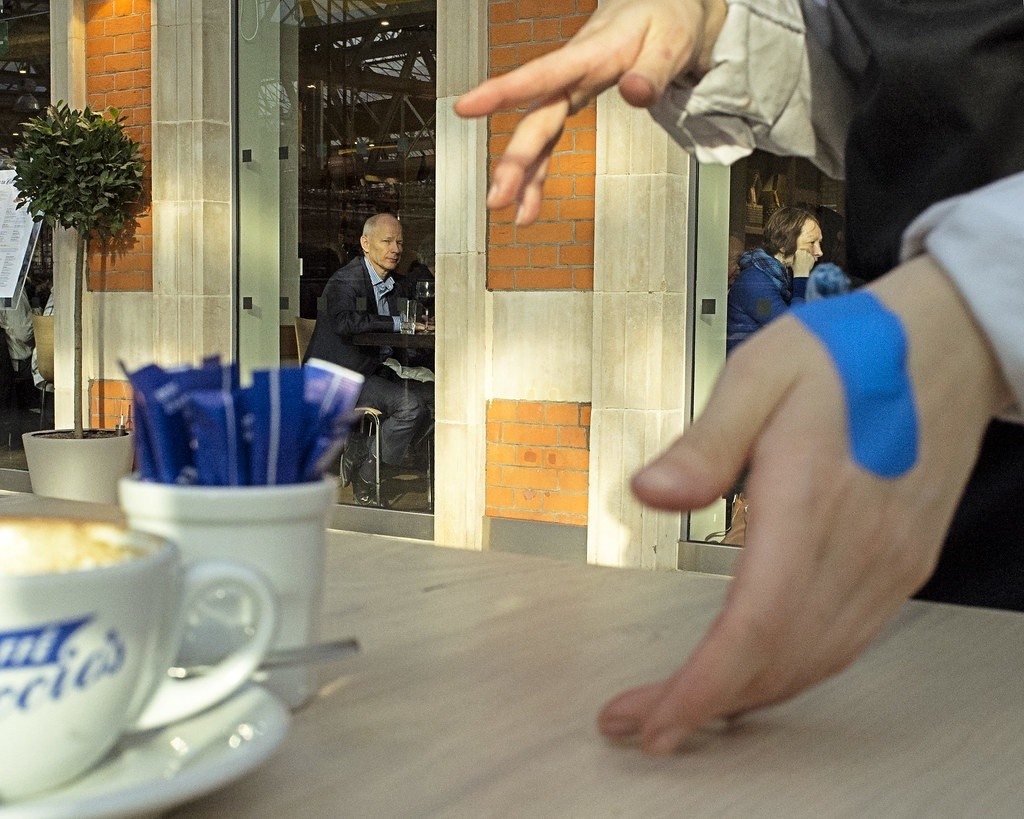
[
  {"left": 340, "top": 433, "right": 372, "bottom": 488},
  {"left": 352, "top": 455, "right": 393, "bottom": 509}
]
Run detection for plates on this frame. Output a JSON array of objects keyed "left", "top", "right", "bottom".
[{"left": 0, "top": 664, "right": 289, "bottom": 819}]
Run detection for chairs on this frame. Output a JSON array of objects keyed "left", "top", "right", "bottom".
[{"left": 292, "top": 317, "right": 384, "bottom": 507}]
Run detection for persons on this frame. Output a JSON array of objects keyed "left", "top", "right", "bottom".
[
  {"left": 301, "top": 213, "right": 434, "bottom": 510},
  {"left": 0, "top": 264, "right": 55, "bottom": 450},
  {"left": 726, "top": 207, "right": 824, "bottom": 360},
  {"left": 454, "top": 0, "right": 1024, "bottom": 757}
]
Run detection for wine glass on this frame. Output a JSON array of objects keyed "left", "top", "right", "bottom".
[{"left": 416, "top": 280, "right": 435, "bottom": 335}]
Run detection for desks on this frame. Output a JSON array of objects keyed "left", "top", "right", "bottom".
[
  {"left": 352, "top": 330, "right": 436, "bottom": 349},
  {"left": 0, "top": 491, "right": 1024, "bottom": 818}
]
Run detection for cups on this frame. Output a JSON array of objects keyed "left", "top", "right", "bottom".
[
  {"left": 118, "top": 472, "right": 336, "bottom": 664},
  {"left": 399, "top": 299, "right": 417, "bottom": 334},
  {"left": 0, "top": 515, "right": 280, "bottom": 800}
]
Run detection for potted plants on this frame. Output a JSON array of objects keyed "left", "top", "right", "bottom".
[{"left": 9, "top": 101, "right": 147, "bottom": 504}]
